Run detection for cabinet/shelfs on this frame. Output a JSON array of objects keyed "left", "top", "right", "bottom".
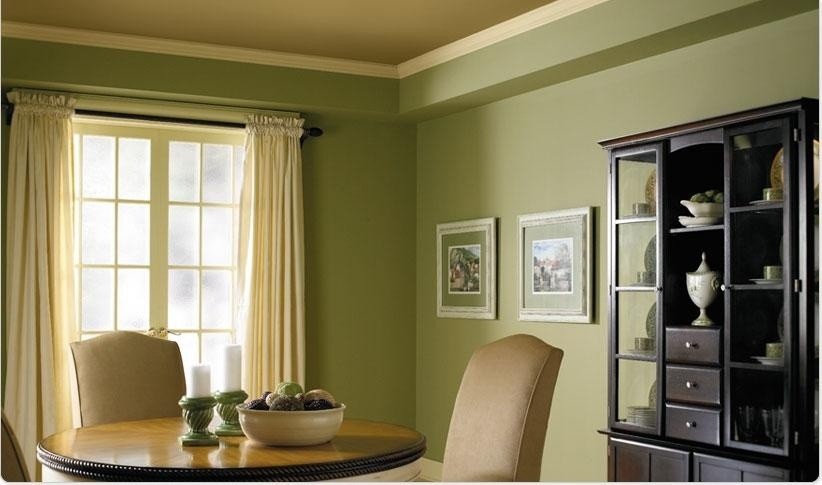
[
  {"left": 597, "top": 95, "right": 820, "bottom": 467},
  {"left": 607, "top": 434, "right": 815, "bottom": 482}
]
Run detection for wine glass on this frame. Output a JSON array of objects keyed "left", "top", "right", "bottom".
[{"left": 737, "top": 404, "right": 786, "bottom": 448}]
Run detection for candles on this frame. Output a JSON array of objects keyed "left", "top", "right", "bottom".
[
  {"left": 219, "top": 344, "right": 242, "bottom": 393},
  {"left": 191, "top": 364, "right": 210, "bottom": 398}
]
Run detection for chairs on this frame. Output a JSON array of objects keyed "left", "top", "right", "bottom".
[
  {"left": 67, "top": 330, "right": 186, "bottom": 427},
  {"left": 441, "top": 333, "right": 564, "bottom": 482},
  {"left": 1, "top": 408, "right": 32, "bottom": 481}
]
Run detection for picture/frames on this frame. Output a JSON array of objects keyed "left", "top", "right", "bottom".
[
  {"left": 517, "top": 205, "right": 593, "bottom": 324},
  {"left": 436, "top": 217, "right": 497, "bottom": 320}
]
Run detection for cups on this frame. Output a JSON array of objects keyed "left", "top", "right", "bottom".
[
  {"left": 763, "top": 186, "right": 784, "bottom": 201},
  {"left": 634, "top": 336, "right": 657, "bottom": 352},
  {"left": 636, "top": 269, "right": 655, "bottom": 285},
  {"left": 766, "top": 340, "right": 786, "bottom": 358},
  {"left": 632, "top": 201, "right": 654, "bottom": 216},
  {"left": 762, "top": 264, "right": 783, "bottom": 279}
]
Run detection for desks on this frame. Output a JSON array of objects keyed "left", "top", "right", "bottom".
[{"left": 36, "top": 411, "right": 428, "bottom": 481}]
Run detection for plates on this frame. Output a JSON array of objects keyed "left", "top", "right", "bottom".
[
  {"left": 622, "top": 212, "right": 656, "bottom": 217},
  {"left": 749, "top": 276, "right": 783, "bottom": 285},
  {"left": 648, "top": 377, "right": 658, "bottom": 408},
  {"left": 748, "top": 198, "right": 783, "bottom": 205},
  {"left": 628, "top": 349, "right": 657, "bottom": 356},
  {"left": 749, "top": 353, "right": 785, "bottom": 367},
  {"left": 644, "top": 166, "right": 657, "bottom": 212},
  {"left": 629, "top": 281, "right": 657, "bottom": 287},
  {"left": 776, "top": 292, "right": 820, "bottom": 359},
  {"left": 626, "top": 403, "right": 657, "bottom": 428},
  {"left": 778, "top": 213, "right": 819, "bottom": 282},
  {"left": 679, "top": 215, "right": 720, "bottom": 228},
  {"left": 647, "top": 301, "right": 659, "bottom": 338},
  {"left": 644, "top": 232, "right": 657, "bottom": 273},
  {"left": 769, "top": 138, "right": 819, "bottom": 202}
]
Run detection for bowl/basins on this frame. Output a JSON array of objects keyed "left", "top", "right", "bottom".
[
  {"left": 680, "top": 199, "right": 724, "bottom": 218},
  {"left": 235, "top": 400, "right": 347, "bottom": 447}
]
